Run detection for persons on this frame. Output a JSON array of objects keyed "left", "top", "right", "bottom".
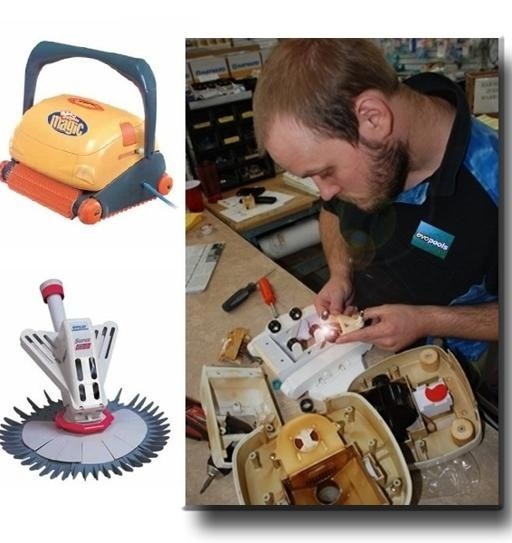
[{"left": 250, "top": 39, "right": 498, "bottom": 397}]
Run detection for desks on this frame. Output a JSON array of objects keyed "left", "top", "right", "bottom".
[
  {"left": 185, "top": 200, "right": 501, "bottom": 507},
  {"left": 188, "top": 174, "right": 326, "bottom": 239}
]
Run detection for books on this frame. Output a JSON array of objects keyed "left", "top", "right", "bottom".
[{"left": 282, "top": 171, "right": 320, "bottom": 198}]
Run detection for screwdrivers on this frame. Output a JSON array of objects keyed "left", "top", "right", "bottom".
[
  {"left": 258, "top": 277, "right": 281, "bottom": 319},
  {"left": 221, "top": 267, "right": 278, "bottom": 313}
]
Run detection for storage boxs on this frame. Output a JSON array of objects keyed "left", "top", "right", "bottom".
[
  {"left": 225, "top": 43, "right": 263, "bottom": 79},
  {"left": 464, "top": 69, "right": 501, "bottom": 119},
  {"left": 187, "top": 47, "right": 230, "bottom": 82}
]
